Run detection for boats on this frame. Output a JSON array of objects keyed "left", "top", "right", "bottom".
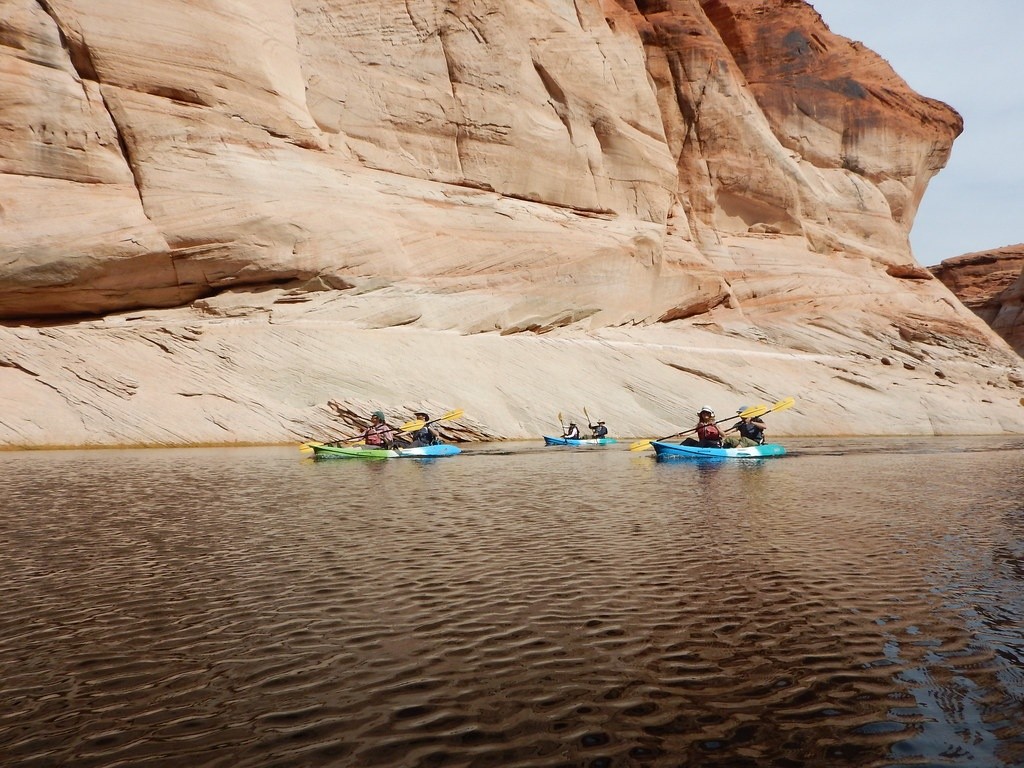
[
  {"left": 308, "top": 441, "right": 461, "bottom": 459},
  {"left": 541, "top": 432, "right": 619, "bottom": 447},
  {"left": 649, "top": 437, "right": 788, "bottom": 462}
]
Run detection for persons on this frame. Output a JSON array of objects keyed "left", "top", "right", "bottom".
[
  {"left": 562, "top": 419, "right": 609, "bottom": 439},
  {"left": 673, "top": 405, "right": 768, "bottom": 449},
  {"left": 345, "top": 408, "right": 440, "bottom": 450}
]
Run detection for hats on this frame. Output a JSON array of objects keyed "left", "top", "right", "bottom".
[
  {"left": 597, "top": 419, "right": 605, "bottom": 424},
  {"left": 413, "top": 410, "right": 429, "bottom": 421},
  {"left": 697, "top": 407, "right": 715, "bottom": 418},
  {"left": 371, "top": 411, "right": 384, "bottom": 421},
  {"left": 736, "top": 406, "right": 748, "bottom": 413},
  {"left": 570, "top": 420, "right": 577, "bottom": 425}
]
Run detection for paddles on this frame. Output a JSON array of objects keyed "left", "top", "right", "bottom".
[
  {"left": 558, "top": 412, "right": 567, "bottom": 445},
  {"left": 299, "top": 419, "right": 424, "bottom": 454},
  {"left": 629, "top": 404, "right": 767, "bottom": 453},
  {"left": 584, "top": 406, "right": 597, "bottom": 439},
  {"left": 425, "top": 408, "right": 463, "bottom": 426},
  {"left": 723, "top": 396, "right": 795, "bottom": 433}
]
